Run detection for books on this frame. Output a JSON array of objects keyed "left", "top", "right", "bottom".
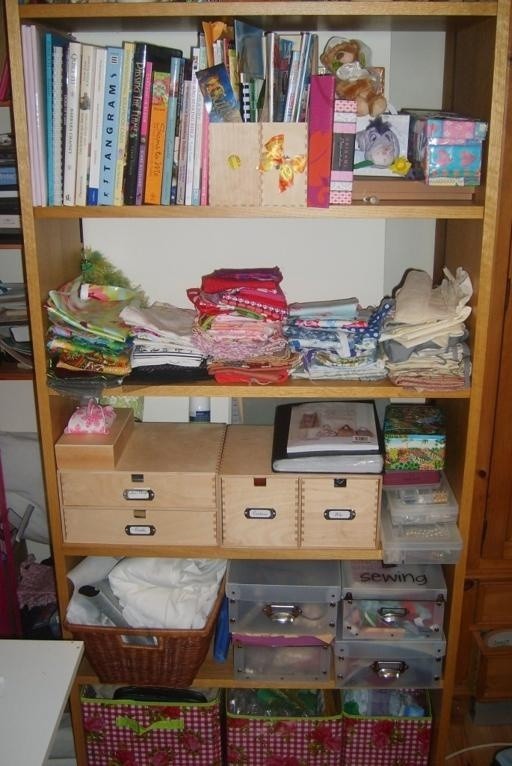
[
  {"left": 270, "top": 401, "right": 386, "bottom": 475},
  {"left": 20, "top": 18, "right": 358, "bottom": 208},
  {"left": 0, "top": 132, "right": 36, "bottom": 370}
]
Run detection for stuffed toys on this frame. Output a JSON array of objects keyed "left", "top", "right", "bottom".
[{"left": 320, "top": 34, "right": 387, "bottom": 116}]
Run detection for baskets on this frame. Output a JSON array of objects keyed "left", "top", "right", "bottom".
[{"left": 61, "top": 566, "right": 229, "bottom": 690}]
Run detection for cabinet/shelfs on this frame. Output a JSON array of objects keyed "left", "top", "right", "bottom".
[{"left": 2, "top": 1, "right": 511, "bottom": 765}]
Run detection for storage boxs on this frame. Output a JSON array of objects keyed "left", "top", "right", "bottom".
[
  {"left": 384, "top": 400, "right": 449, "bottom": 474},
  {"left": 220, "top": 424, "right": 381, "bottom": 546},
  {"left": 381, "top": 470, "right": 463, "bottom": 564},
  {"left": 224, "top": 688, "right": 342, "bottom": 766},
  {"left": 205, "top": 78, "right": 308, "bottom": 212},
  {"left": 61, "top": 422, "right": 227, "bottom": 542},
  {"left": 82, "top": 682, "right": 223, "bottom": 764},
  {"left": 352, "top": 111, "right": 409, "bottom": 177},
  {"left": 401, "top": 107, "right": 490, "bottom": 188},
  {"left": 224, "top": 560, "right": 342, "bottom": 636},
  {"left": 56, "top": 403, "right": 133, "bottom": 470},
  {"left": 232, "top": 640, "right": 334, "bottom": 682},
  {"left": 334, "top": 615, "right": 444, "bottom": 681},
  {"left": 340, "top": 559, "right": 450, "bottom": 641},
  {"left": 342, "top": 688, "right": 433, "bottom": 765}
]
